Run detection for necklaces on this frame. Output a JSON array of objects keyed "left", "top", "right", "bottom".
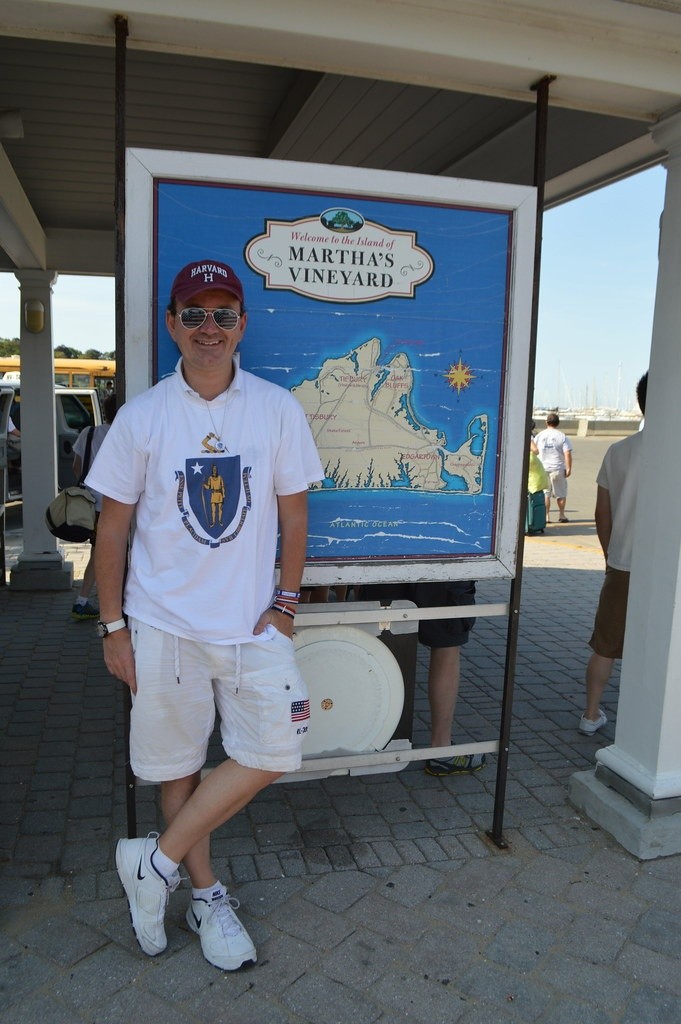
[{"left": 206, "top": 390, "right": 229, "bottom": 451}]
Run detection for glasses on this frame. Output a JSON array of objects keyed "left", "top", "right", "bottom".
[{"left": 174, "top": 307, "right": 241, "bottom": 332}]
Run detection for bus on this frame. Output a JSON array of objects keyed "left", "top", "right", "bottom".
[{"left": 0, "top": 357, "right": 115, "bottom": 425}]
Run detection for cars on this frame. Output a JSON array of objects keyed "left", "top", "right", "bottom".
[{"left": 0, "top": 378, "right": 104, "bottom": 587}]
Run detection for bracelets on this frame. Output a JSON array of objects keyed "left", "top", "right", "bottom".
[
  {"left": 274, "top": 596, "right": 299, "bottom": 604},
  {"left": 276, "top": 590, "right": 300, "bottom": 598},
  {"left": 270, "top": 602, "right": 295, "bottom": 619}
]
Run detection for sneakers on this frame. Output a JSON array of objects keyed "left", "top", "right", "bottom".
[
  {"left": 578, "top": 708, "right": 606, "bottom": 736},
  {"left": 185, "top": 886, "right": 257, "bottom": 971},
  {"left": 115, "top": 831, "right": 189, "bottom": 956},
  {"left": 425, "top": 741, "right": 486, "bottom": 777},
  {"left": 72, "top": 601, "right": 99, "bottom": 619}
]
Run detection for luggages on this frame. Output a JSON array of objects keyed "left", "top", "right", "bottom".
[{"left": 525, "top": 490, "right": 546, "bottom": 535}]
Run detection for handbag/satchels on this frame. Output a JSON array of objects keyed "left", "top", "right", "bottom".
[
  {"left": 44, "top": 486, "right": 97, "bottom": 542},
  {"left": 528, "top": 451, "right": 548, "bottom": 494}
]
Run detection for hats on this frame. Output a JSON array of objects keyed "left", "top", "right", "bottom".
[{"left": 170, "top": 260, "right": 243, "bottom": 302}]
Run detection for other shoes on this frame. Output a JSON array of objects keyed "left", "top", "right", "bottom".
[{"left": 558, "top": 518, "right": 567, "bottom": 522}]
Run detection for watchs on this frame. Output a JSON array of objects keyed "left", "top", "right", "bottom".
[{"left": 98, "top": 618, "right": 126, "bottom": 638}]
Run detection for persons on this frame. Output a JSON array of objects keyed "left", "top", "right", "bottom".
[
  {"left": 82, "top": 261, "right": 326, "bottom": 972},
  {"left": 530, "top": 422, "right": 539, "bottom": 455},
  {"left": 578, "top": 370, "right": 648, "bottom": 735},
  {"left": 70, "top": 394, "right": 115, "bottom": 621},
  {"left": 8, "top": 416, "right": 20, "bottom": 437},
  {"left": 299, "top": 585, "right": 352, "bottom": 604},
  {"left": 104, "top": 382, "right": 113, "bottom": 397},
  {"left": 360, "top": 580, "right": 486, "bottom": 776},
  {"left": 534, "top": 413, "right": 573, "bottom": 523}
]
